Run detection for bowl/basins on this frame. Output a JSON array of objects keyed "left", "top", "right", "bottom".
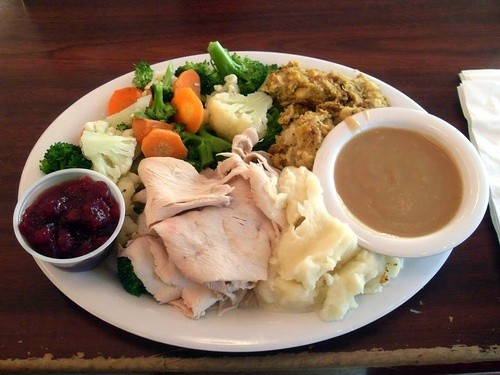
[
  {"left": 13, "top": 169, "right": 126, "bottom": 272},
  {"left": 311, "top": 106, "right": 489, "bottom": 258}
]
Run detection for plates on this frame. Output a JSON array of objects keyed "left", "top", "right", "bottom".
[{"left": 16, "top": 52, "right": 453, "bottom": 352}]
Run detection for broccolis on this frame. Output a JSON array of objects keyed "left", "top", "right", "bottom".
[{"left": 39, "top": 40, "right": 279, "bottom": 184}]
